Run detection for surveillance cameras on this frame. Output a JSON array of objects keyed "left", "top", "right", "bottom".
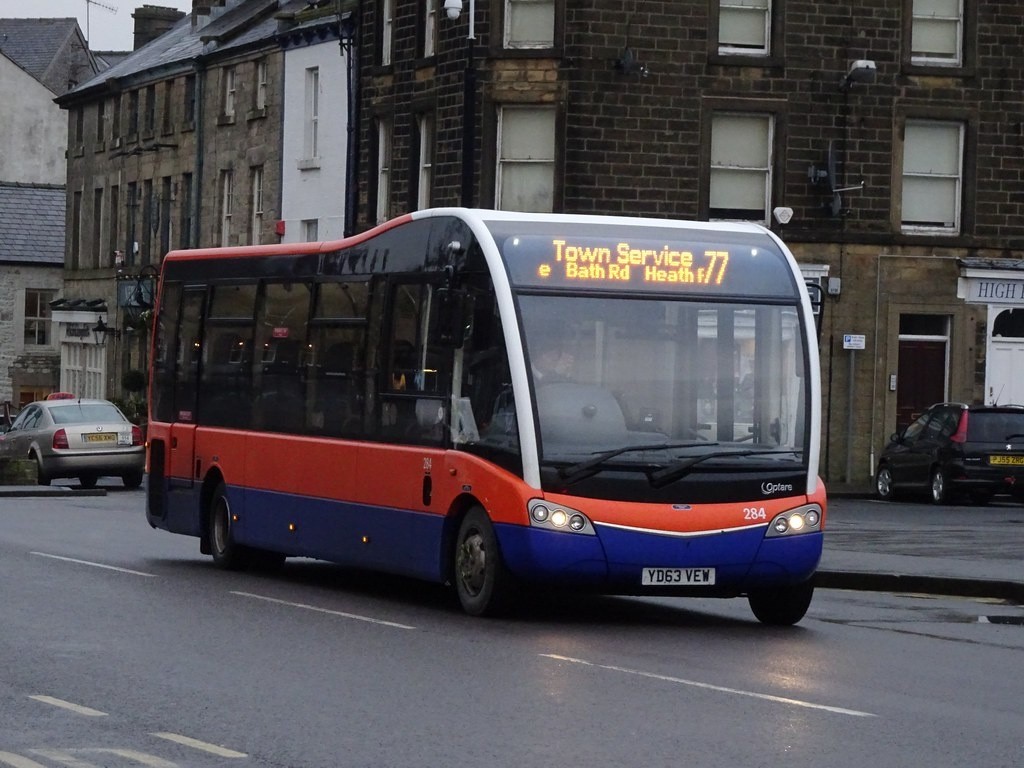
[{"left": 441, "top": 1, "right": 465, "bottom": 19}]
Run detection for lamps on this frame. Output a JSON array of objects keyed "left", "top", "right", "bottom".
[
  {"left": 840, "top": 60, "right": 877, "bottom": 89},
  {"left": 49, "top": 298, "right": 67, "bottom": 307},
  {"left": 142, "top": 143, "right": 178, "bottom": 152},
  {"left": 92, "top": 315, "right": 122, "bottom": 346},
  {"left": 86, "top": 299, "right": 105, "bottom": 308},
  {"left": 108, "top": 151, "right": 140, "bottom": 161},
  {"left": 68, "top": 299, "right": 85, "bottom": 307},
  {"left": 129, "top": 145, "right": 159, "bottom": 157}
]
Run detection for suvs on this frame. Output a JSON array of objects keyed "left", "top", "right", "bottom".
[{"left": 874, "top": 401, "right": 1024, "bottom": 505}]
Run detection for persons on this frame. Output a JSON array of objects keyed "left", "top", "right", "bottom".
[{"left": 530, "top": 344, "right": 563, "bottom": 384}]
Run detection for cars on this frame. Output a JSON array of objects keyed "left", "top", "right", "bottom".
[{"left": 0, "top": 398, "right": 145, "bottom": 489}]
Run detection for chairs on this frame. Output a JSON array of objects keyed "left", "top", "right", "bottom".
[{"left": 160, "top": 374, "right": 370, "bottom": 435}]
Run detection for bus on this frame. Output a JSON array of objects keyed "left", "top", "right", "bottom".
[{"left": 146, "top": 206, "right": 828, "bottom": 626}]
